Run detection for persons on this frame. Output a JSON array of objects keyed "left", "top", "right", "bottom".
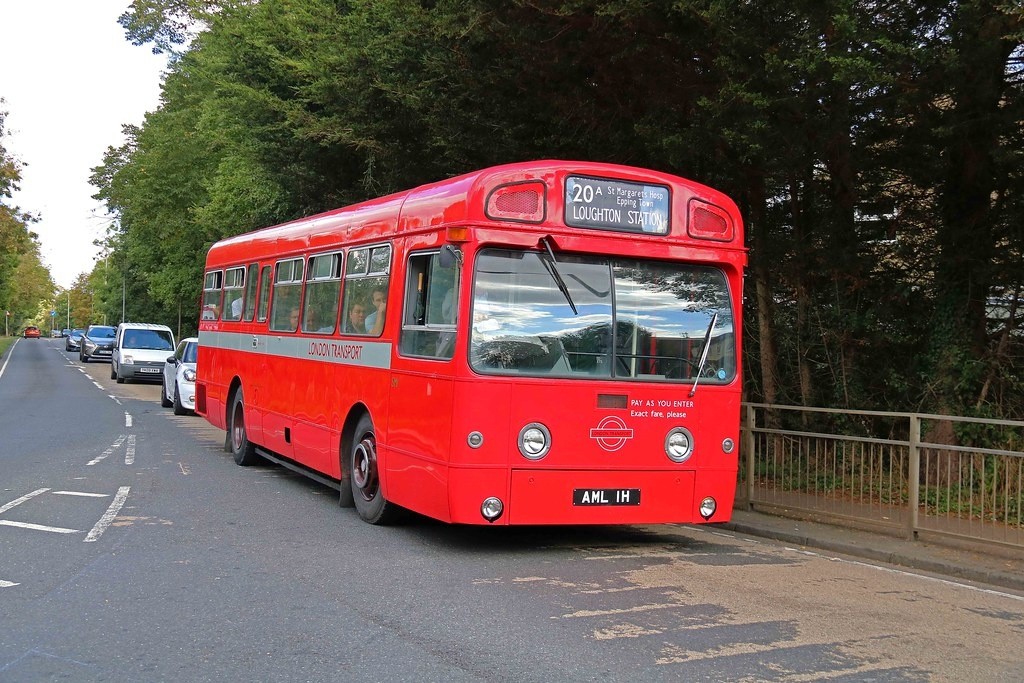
[
  {"left": 231, "top": 290, "right": 249, "bottom": 320},
  {"left": 288, "top": 285, "right": 387, "bottom": 335},
  {"left": 438, "top": 274, "right": 496, "bottom": 356},
  {"left": 123, "top": 336, "right": 139, "bottom": 348}
]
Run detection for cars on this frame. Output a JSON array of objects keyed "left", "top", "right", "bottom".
[
  {"left": 160, "top": 336, "right": 200, "bottom": 415},
  {"left": 66, "top": 328, "right": 87, "bottom": 352},
  {"left": 63, "top": 328, "right": 71, "bottom": 337},
  {"left": 51, "top": 330, "right": 63, "bottom": 338}
]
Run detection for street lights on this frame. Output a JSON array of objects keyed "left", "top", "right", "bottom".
[
  {"left": 67, "top": 293, "right": 69, "bottom": 329},
  {"left": 101, "top": 258, "right": 107, "bottom": 326}
]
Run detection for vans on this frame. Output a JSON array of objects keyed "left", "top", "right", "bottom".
[{"left": 110, "top": 322, "right": 178, "bottom": 384}]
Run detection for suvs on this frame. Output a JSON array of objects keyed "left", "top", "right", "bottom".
[
  {"left": 24, "top": 326, "right": 41, "bottom": 339},
  {"left": 78, "top": 325, "right": 118, "bottom": 363}
]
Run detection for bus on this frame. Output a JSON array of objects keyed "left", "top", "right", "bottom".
[{"left": 191, "top": 158, "right": 753, "bottom": 527}]
